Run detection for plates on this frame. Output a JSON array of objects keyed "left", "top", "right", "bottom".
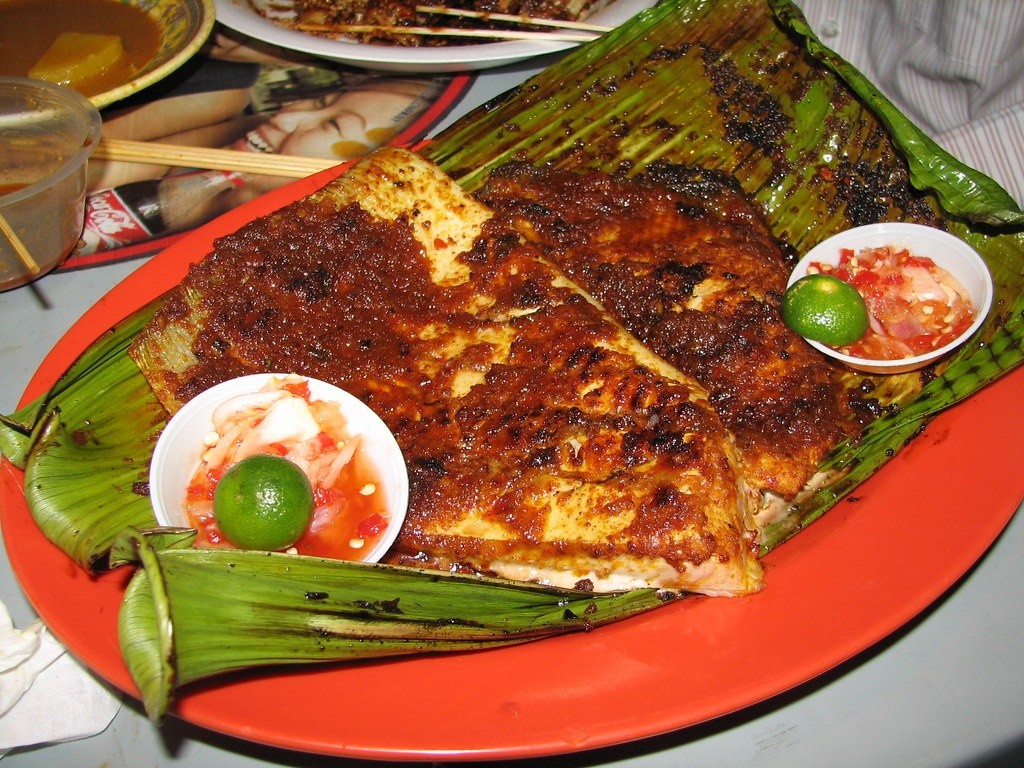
[
  {"left": 2, "top": 137, "right": 1024, "bottom": 761},
  {"left": 1, "top": 0, "right": 215, "bottom": 129},
  {"left": 215, "top": 0, "right": 660, "bottom": 75}
]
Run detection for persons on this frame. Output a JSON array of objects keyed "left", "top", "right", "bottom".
[
  {"left": 80, "top": 19, "right": 326, "bottom": 124},
  {"left": 87, "top": 79, "right": 449, "bottom": 237}
]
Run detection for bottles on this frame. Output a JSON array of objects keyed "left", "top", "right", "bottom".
[{"left": 64, "top": 169, "right": 253, "bottom": 259}]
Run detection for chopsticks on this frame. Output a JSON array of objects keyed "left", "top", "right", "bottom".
[{"left": 296, "top": 6, "right": 615, "bottom": 43}]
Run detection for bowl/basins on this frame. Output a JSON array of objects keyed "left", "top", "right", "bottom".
[{"left": 0, "top": 77, "right": 105, "bottom": 288}]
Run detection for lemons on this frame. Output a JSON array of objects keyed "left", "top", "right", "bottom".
[
  {"left": 778, "top": 273, "right": 868, "bottom": 346},
  {"left": 213, "top": 455, "right": 313, "bottom": 552}
]
